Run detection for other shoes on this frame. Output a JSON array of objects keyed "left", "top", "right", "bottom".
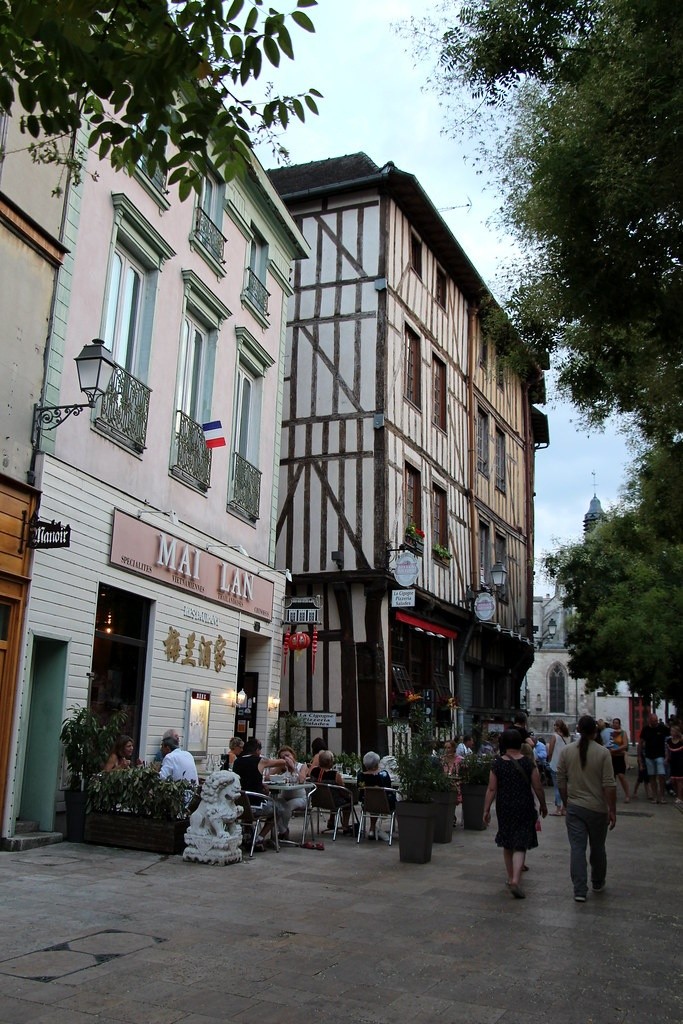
[
  {"left": 343, "top": 828, "right": 354, "bottom": 834},
  {"left": 651, "top": 798, "right": 657, "bottom": 803},
  {"left": 278, "top": 827, "right": 289, "bottom": 847},
  {"left": 647, "top": 793, "right": 652, "bottom": 798},
  {"left": 392, "top": 832, "right": 399, "bottom": 838},
  {"left": 522, "top": 864, "right": 529, "bottom": 871},
  {"left": 505, "top": 881, "right": 512, "bottom": 888},
  {"left": 549, "top": 811, "right": 562, "bottom": 816},
  {"left": 592, "top": 881, "right": 606, "bottom": 892},
  {"left": 574, "top": 894, "right": 586, "bottom": 901},
  {"left": 561, "top": 809, "right": 566, "bottom": 815},
  {"left": 368, "top": 831, "right": 375, "bottom": 839},
  {"left": 327, "top": 820, "right": 335, "bottom": 829},
  {"left": 624, "top": 796, "right": 630, "bottom": 802},
  {"left": 254, "top": 839, "right": 264, "bottom": 846},
  {"left": 661, "top": 799, "right": 666, "bottom": 803},
  {"left": 632, "top": 794, "right": 637, "bottom": 798},
  {"left": 510, "top": 883, "right": 525, "bottom": 898}
]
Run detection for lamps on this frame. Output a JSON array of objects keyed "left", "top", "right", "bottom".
[
  {"left": 514, "top": 618, "right": 526, "bottom": 627},
  {"left": 332, "top": 550, "right": 344, "bottom": 573},
  {"left": 535, "top": 619, "right": 557, "bottom": 640},
  {"left": 389, "top": 540, "right": 425, "bottom": 558},
  {"left": 480, "top": 559, "right": 508, "bottom": 597},
  {"left": 268, "top": 695, "right": 281, "bottom": 711},
  {"left": 232, "top": 688, "right": 246, "bottom": 708},
  {"left": 530, "top": 626, "right": 539, "bottom": 635},
  {"left": 32, "top": 338, "right": 293, "bottom": 583},
  {"left": 459, "top": 591, "right": 477, "bottom": 603}
]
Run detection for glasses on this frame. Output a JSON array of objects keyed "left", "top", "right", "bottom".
[{"left": 554, "top": 724, "right": 556, "bottom": 726}]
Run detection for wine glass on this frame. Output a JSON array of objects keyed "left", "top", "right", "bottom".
[
  {"left": 346, "top": 767, "right": 351, "bottom": 775},
  {"left": 206, "top": 753, "right": 213, "bottom": 771},
  {"left": 354, "top": 764, "right": 360, "bottom": 779}
]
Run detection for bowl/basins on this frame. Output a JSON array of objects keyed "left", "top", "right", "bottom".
[{"left": 270, "top": 775, "right": 284, "bottom": 782}]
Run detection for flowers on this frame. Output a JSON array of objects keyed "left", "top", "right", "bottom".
[
  {"left": 446, "top": 697, "right": 462, "bottom": 709},
  {"left": 407, "top": 523, "right": 425, "bottom": 538},
  {"left": 404, "top": 689, "right": 421, "bottom": 702}
]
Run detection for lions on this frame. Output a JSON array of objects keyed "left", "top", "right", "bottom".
[{"left": 186, "top": 770, "right": 244, "bottom": 839}]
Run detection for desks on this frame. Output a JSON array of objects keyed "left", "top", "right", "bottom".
[
  {"left": 341, "top": 776, "right": 401, "bottom": 837},
  {"left": 264, "top": 783, "right": 313, "bottom": 850}
]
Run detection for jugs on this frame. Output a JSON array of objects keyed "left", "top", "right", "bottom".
[{"left": 333, "top": 763, "right": 343, "bottom": 778}]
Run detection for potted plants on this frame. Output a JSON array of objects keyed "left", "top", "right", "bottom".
[
  {"left": 379, "top": 696, "right": 437, "bottom": 864},
  {"left": 458, "top": 716, "right": 498, "bottom": 831},
  {"left": 60, "top": 701, "right": 200, "bottom": 855},
  {"left": 435, "top": 758, "right": 458, "bottom": 843},
  {"left": 432, "top": 545, "right": 453, "bottom": 564}
]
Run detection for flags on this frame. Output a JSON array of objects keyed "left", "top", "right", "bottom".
[{"left": 203, "top": 421, "right": 226, "bottom": 449}]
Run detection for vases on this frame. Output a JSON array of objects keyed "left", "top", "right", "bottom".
[{"left": 406, "top": 534, "right": 414, "bottom": 544}]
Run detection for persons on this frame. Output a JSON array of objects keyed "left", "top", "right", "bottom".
[
  {"left": 160, "top": 737, "right": 198, "bottom": 806},
  {"left": 634, "top": 713, "right": 683, "bottom": 803},
  {"left": 478, "top": 732, "right": 498, "bottom": 757},
  {"left": 531, "top": 735, "right": 547, "bottom": 772},
  {"left": 310, "top": 751, "right": 353, "bottom": 834},
  {"left": 556, "top": 716, "right": 616, "bottom": 902},
  {"left": 309, "top": 738, "right": 327, "bottom": 767},
  {"left": 548, "top": 720, "right": 571, "bottom": 816},
  {"left": 233, "top": 738, "right": 286, "bottom": 851},
  {"left": 104, "top": 735, "right": 135, "bottom": 770},
  {"left": 228, "top": 737, "right": 244, "bottom": 767},
  {"left": 153, "top": 729, "right": 179, "bottom": 763},
  {"left": 595, "top": 718, "right": 631, "bottom": 802},
  {"left": 483, "top": 730, "right": 548, "bottom": 898},
  {"left": 426, "top": 740, "right": 465, "bottom": 826},
  {"left": 509, "top": 712, "right": 535, "bottom": 746},
  {"left": 455, "top": 735, "right": 473, "bottom": 757},
  {"left": 357, "top": 751, "right": 396, "bottom": 841},
  {"left": 269, "top": 746, "right": 309, "bottom": 840}
]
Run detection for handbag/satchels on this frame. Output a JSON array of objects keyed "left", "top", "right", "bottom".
[{"left": 535, "top": 820, "right": 541, "bottom": 832}]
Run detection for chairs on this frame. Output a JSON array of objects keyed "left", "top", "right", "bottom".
[{"left": 238, "top": 782, "right": 398, "bottom": 857}]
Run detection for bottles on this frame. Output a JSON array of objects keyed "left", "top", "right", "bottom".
[
  {"left": 220, "top": 748, "right": 229, "bottom": 770},
  {"left": 452, "top": 763, "right": 456, "bottom": 776}
]
